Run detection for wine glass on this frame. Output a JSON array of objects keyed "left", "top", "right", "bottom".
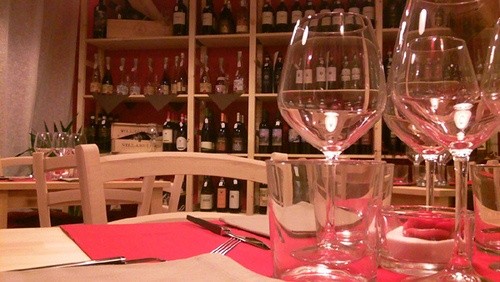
[
  {"left": 275, "top": 12, "right": 387, "bottom": 264},
  {"left": 389, "top": 0, "right": 500, "bottom": 281},
  {"left": 382, "top": 35, "right": 481, "bottom": 216},
  {"left": 33, "top": 132, "right": 88, "bottom": 180}
]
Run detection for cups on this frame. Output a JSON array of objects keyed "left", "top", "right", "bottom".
[
  {"left": 265, "top": 158, "right": 386, "bottom": 282},
  {"left": 466, "top": 162, "right": 500, "bottom": 254},
  {"left": 412, "top": 163, "right": 433, "bottom": 188},
  {"left": 392, "top": 163, "right": 408, "bottom": 184},
  {"left": 445, "top": 164, "right": 466, "bottom": 188},
  {"left": 306, "top": 159, "right": 395, "bottom": 266}
]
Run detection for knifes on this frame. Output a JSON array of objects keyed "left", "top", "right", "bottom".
[{"left": 185, "top": 214, "right": 273, "bottom": 251}]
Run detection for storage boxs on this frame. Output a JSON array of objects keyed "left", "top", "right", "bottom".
[
  {"left": 106, "top": 18, "right": 172, "bottom": 40},
  {"left": 110, "top": 122, "right": 164, "bottom": 155}
]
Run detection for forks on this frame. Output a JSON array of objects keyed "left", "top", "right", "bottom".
[{"left": 210, "top": 237, "right": 241, "bottom": 256}]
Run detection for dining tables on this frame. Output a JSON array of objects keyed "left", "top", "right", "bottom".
[
  {"left": 0, "top": 227, "right": 500, "bottom": 282},
  {"left": 0, "top": 179, "right": 171, "bottom": 229}
]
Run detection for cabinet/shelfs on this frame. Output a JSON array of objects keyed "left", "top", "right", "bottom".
[{"left": 74, "top": 0, "right": 500, "bottom": 215}]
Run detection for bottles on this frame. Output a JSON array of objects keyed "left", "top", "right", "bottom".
[
  {"left": 255, "top": 181, "right": 268, "bottom": 215},
  {"left": 201, "top": 0, "right": 250, "bottom": 33},
  {"left": 161, "top": 111, "right": 187, "bottom": 151},
  {"left": 199, "top": 111, "right": 247, "bottom": 153},
  {"left": 90, "top": 52, "right": 186, "bottom": 97},
  {"left": 261, "top": 1, "right": 483, "bottom": 32},
  {"left": 262, "top": 46, "right": 495, "bottom": 94},
  {"left": 92, "top": 0, "right": 108, "bottom": 38},
  {"left": 258, "top": 108, "right": 499, "bottom": 155},
  {"left": 172, "top": 0, "right": 188, "bottom": 36},
  {"left": 199, "top": 50, "right": 245, "bottom": 94},
  {"left": 198, "top": 176, "right": 242, "bottom": 210},
  {"left": 87, "top": 110, "right": 119, "bottom": 152}
]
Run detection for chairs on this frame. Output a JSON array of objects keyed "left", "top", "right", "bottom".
[
  {"left": 31, "top": 151, "right": 156, "bottom": 230},
  {"left": 76, "top": 143, "right": 294, "bottom": 225},
  {"left": 0, "top": 156, "right": 37, "bottom": 229},
  {"left": 163, "top": 174, "right": 186, "bottom": 212}
]
